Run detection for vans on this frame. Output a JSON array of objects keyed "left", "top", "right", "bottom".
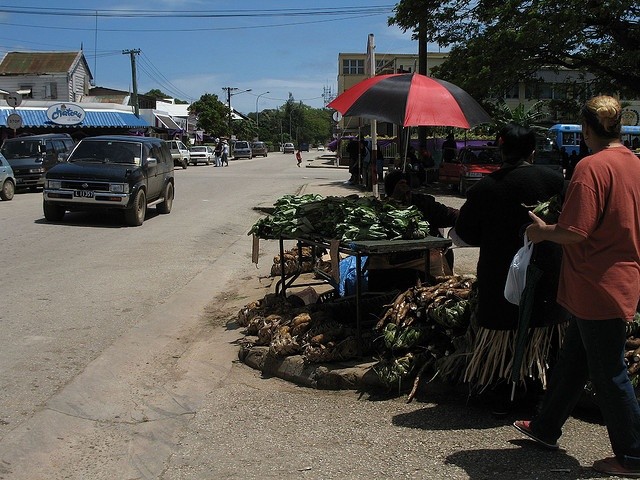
[
  {"left": 3, "top": 133, "right": 75, "bottom": 194},
  {"left": 317, "top": 144, "right": 324, "bottom": 151},
  {"left": 0, "top": 152, "right": 16, "bottom": 201}
]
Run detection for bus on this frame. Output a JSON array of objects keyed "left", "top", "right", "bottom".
[{"left": 547, "top": 124, "right": 640, "bottom": 159}]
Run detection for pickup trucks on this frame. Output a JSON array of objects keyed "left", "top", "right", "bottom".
[
  {"left": 439, "top": 145, "right": 503, "bottom": 197},
  {"left": 164, "top": 140, "right": 190, "bottom": 169}
]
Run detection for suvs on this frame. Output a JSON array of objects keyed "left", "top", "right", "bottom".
[
  {"left": 234, "top": 141, "right": 252, "bottom": 159},
  {"left": 252, "top": 142, "right": 267, "bottom": 157},
  {"left": 43, "top": 135, "right": 175, "bottom": 226}
]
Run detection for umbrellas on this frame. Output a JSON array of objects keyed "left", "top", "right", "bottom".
[{"left": 326, "top": 58, "right": 495, "bottom": 181}]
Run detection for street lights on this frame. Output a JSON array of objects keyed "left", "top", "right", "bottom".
[
  {"left": 256, "top": 91, "right": 270, "bottom": 126},
  {"left": 230, "top": 89, "right": 252, "bottom": 96}
]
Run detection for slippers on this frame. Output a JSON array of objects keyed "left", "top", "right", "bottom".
[
  {"left": 513, "top": 421, "right": 559, "bottom": 449},
  {"left": 592, "top": 457, "right": 640, "bottom": 476}
]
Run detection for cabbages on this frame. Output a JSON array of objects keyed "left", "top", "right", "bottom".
[
  {"left": 625, "top": 312, "right": 640, "bottom": 336},
  {"left": 374, "top": 298, "right": 471, "bottom": 390},
  {"left": 253, "top": 192, "right": 430, "bottom": 239},
  {"left": 533, "top": 193, "right": 564, "bottom": 226}
]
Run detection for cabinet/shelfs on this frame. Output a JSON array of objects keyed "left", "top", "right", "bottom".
[{"left": 277, "top": 230, "right": 452, "bottom": 335}]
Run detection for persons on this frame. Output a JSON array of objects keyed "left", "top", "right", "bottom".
[
  {"left": 361, "top": 169, "right": 460, "bottom": 298},
  {"left": 453, "top": 120, "right": 569, "bottom": 416},
  {"left": 211, "top": 137, "right": 229, "bottom": 167},
  {"left": 347, "top": 138, "right": 384, "bottom": 186},
  {"left": 408, "top": 144, "right": 437, "bottom": 168},
  {"left": 511, "top": 95, "right": 640, "bottom": 477},
  {"left": 296, "top": 149, "right": 303, "bottom": 168}
]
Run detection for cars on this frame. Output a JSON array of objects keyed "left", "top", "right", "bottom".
[
  {"left": 284, "top": 143, "right": 295, "bottom": 154},
  {"left": 190, "top": 146, "right": 216, "bottom": 165},
  {"left": 301, "top": 142, "right": 309, "bottom": 152}
]
